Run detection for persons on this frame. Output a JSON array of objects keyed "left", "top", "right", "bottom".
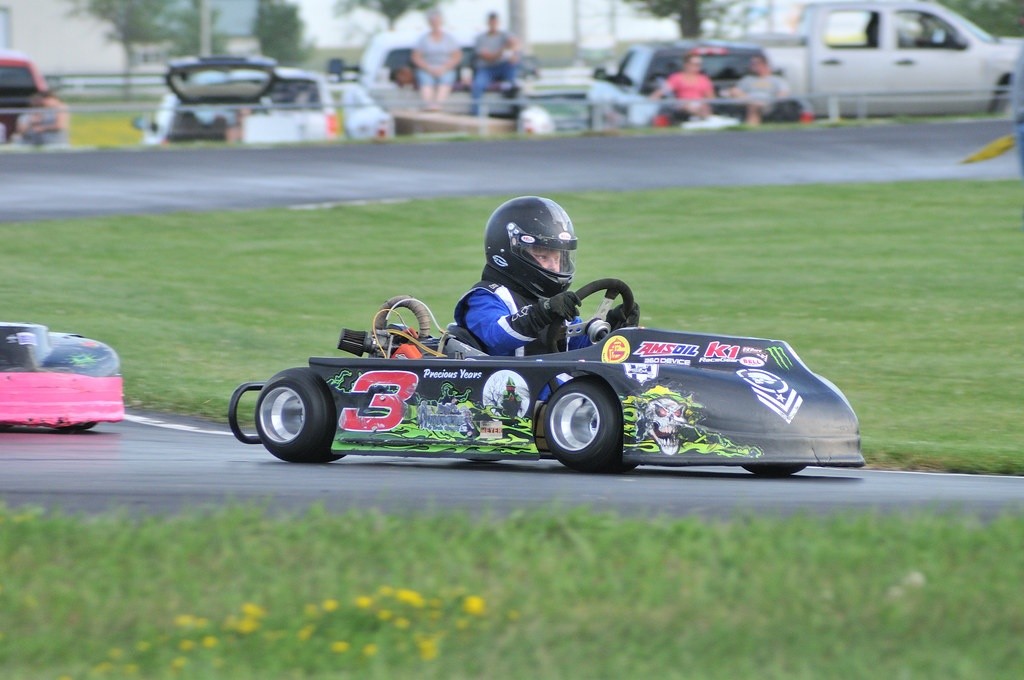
[
  {"left": 22, "top": 86, "right": 71, "bottom": 151},
  {"left": 650, "top": 51, "right": 803, "bottom": 125},
  {"left": 452, "top": 195, "right": 643, "bottom": 407},
  {"left": 410, "top": 6, "right": 530, "bottom": 122}
]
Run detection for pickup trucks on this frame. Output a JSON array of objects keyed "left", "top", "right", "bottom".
[
  {"left": 706, "top": 1, "right": 1024, "bottom": 117},
  {"left": 328, "top": 29, "right": 557, "bottom": 141},
  {"left": 581, "top": 40, "right": 816, "bottom": 132}
]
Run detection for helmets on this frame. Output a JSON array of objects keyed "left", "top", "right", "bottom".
[{"left": 484, "top": 196, "right": 578, "bottom": 300}]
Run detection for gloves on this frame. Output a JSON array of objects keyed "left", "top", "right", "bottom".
[
  {"left": 545, "top": 291, "right": 581, "bottom": 321},
  {"left": 609, "top": 302, "right": 641, "bottom": 328}
]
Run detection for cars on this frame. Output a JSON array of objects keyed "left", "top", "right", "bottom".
[
  {"left": 326, "top": 82, "right": 397, "bottom": 142},
  {"left": 0, "top": 49, "right": 68, "bottom": 146},
  {"left": 133, "top": 54, "right": 336, "bottom": 146}
]
